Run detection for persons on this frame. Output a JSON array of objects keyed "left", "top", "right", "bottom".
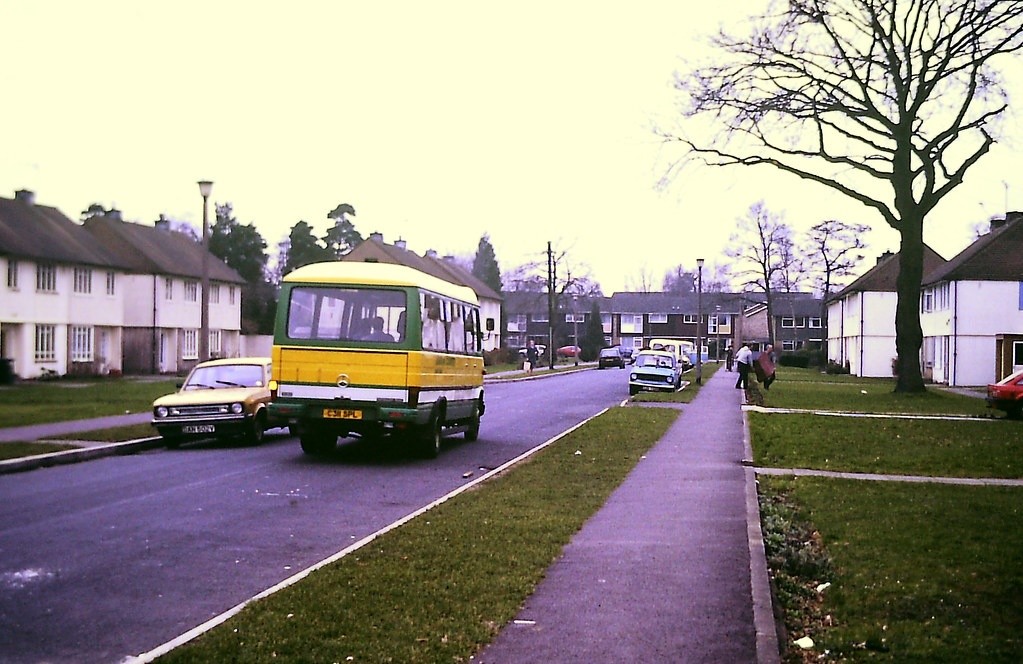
[
  {"left": 722, "top": 345, "right": 735, "bottom": 371},
  {"left": 362, "top": 317, "right": 395, "bottom": 344},
  {"left": 764, "top": 345, "right": 776, "bottom": 390},
  {"left": 352, "top": 318, "right": 372, "bottom": 342},
  {"left": 397, "top": 311, "right": 407, "bottom": 342},
  {"left": 526, "top": 340, "right": 539, "bottom": 373},
  {"left": 733, "top": 342, "right": 753, "bottom": 389}
]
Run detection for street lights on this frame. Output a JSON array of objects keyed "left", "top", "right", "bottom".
[
  {"left": 197, "top": 179, "right": 214, "bottom": 363},
  {"left": 573, "top": 292, "right": 580, "bottom": 366},
  {"left": 695, "top": 257, "right": 705, "bottom": 389},
  {"left": 716, "top": 305, "right": 722, "bottom": 364}
]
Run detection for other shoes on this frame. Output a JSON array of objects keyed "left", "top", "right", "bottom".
[{"left": 736, "top": 386, "right": 742, "bottom": 389}]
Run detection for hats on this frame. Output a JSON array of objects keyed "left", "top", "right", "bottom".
[
  {"left": 767, "top": 344, "right": 772, "bottom": 348},
  {"left": 746, "top": 342, "right": 753, "bottom": 345}
]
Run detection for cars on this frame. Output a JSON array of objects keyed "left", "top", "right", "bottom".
[
  {"left": 631, "top": 346, "right": 646, "bottom": 363},
  {"left": 598, "top": 347, "right": 626, "bottom": 370},
  {"left": 518, "top": 344, "right": 546, "bottom": 360},
  {"left": 556, "top": 346, "right": 583, "bottom": 357},
  {"left": 152, "top": 356, "right": 296, "bottom": 447},
  {"left": 985, "top": 368, "right": 1023, "bottom": 420}
]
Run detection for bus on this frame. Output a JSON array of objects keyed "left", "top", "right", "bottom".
[
  {"left": 649, "top": 339, "right": 708, "bottom": 372},
  {"left": 266, "top": 259, "right": 494, "bottom": 459}
]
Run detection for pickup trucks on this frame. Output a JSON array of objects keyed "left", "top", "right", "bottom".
[{"left": 627, "top": 349, "right": 683, "bottom": 398}]
